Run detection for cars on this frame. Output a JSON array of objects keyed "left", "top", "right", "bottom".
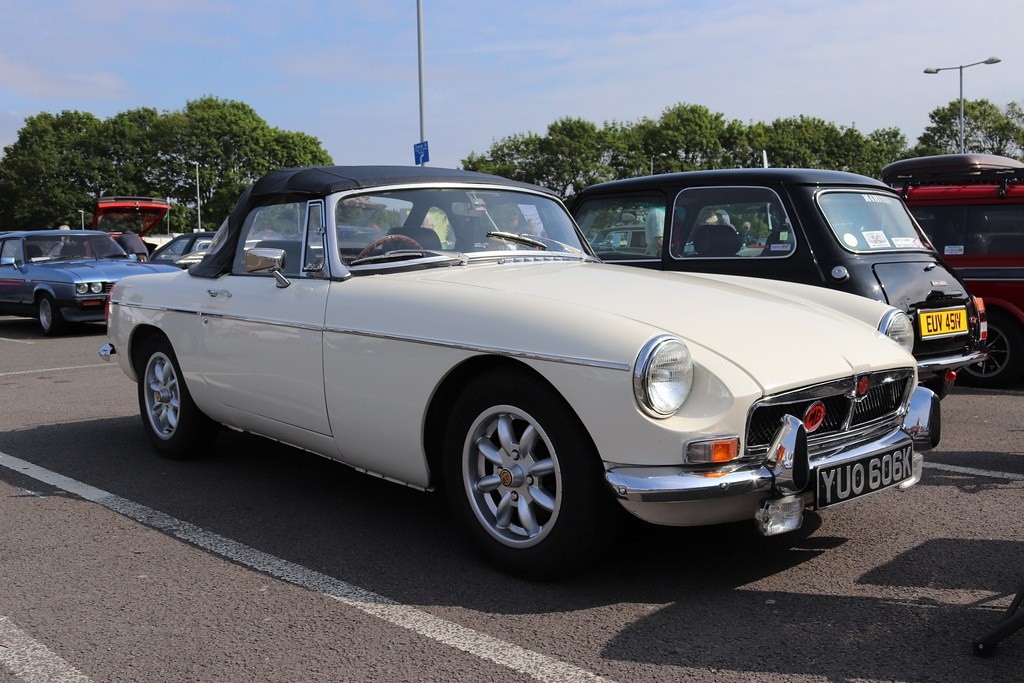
[
  {"left": 148, "top": 230, "right": 219, "bottom": 267},
  {"left": 104, "top": 161, "right": 946, "bottom": 569},
  {"left": 87, "top": 196, "right": 172, "bottom": 258},
  {"left": 552, "top": 164, "right": 997, "bottom": 402},
  {"left": 592, "top": 225, "right": 650, "bottom": 252},
  {"left": 1, "top": 229, "right": 186, "bottom": 335}
]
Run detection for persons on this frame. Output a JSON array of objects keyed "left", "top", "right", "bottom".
[
  {"left": 738, "top": 222, "right": 755, "bottom": 245},
  {"left": 116, "top": 227, "right": 149, "bottom": 263},
  {"left": 474, "top": 201, "right": 541, "bottom": 255},
  {"left": 48, "top": 224, "right": 72, "bottom": 255},
  {"left": 644, "top": 204, "right": 666, "bottom": 255},
  {"left": 527, "top": 218, "right": 537, "bottom": 236}
]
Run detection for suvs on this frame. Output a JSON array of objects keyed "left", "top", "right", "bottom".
[{"left": 882, "top": 154, "right": 1023, "bottom": 381}]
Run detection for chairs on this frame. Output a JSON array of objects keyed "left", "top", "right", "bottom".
[
  {"left": 759, "top": 227, "right": 795, "bottom": 256},
  {"left": 14, "top": 245, "right": 43, "bottom": 261},
  {"left": 694, "top": 225, "right": 743, "bottom": 256},
  {"left": 60, "top": 243, "right": 87, "bottom": 257},
  {"left": 255, "top": 240, "right": 321, "bottom": 275},
  {"left": 381, "top": 227, "right": 443, "bottom": 255}
]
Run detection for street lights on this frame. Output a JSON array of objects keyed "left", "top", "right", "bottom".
[
  {"left": 187, "top": 158, "right": 211, "bottom": 236},
  {"left": 923, "top": 56, "right": 1001, "bottom": 154},
  {"left": 77, "top": 209, "right": 88, "bottom": 231}
]
